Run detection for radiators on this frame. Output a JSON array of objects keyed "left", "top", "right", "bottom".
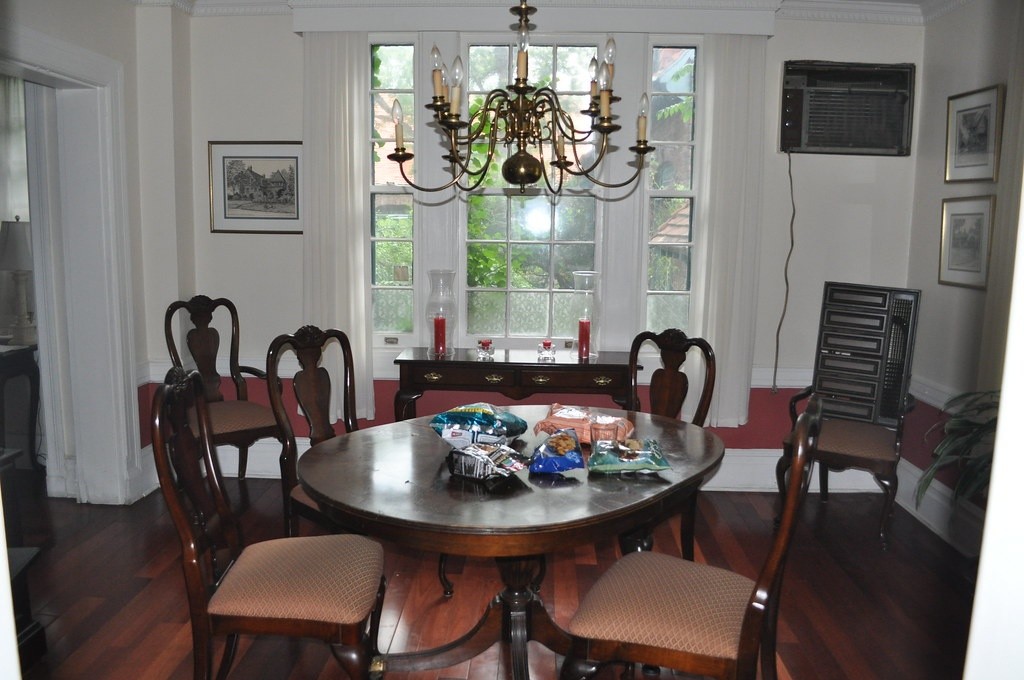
[{"left": 781, "top": 61, "right": 914, "bottom": 155}]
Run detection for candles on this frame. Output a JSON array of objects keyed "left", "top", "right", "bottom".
[
  {"left": 434, "top": 307, "right": 445, "bottom": 355},
  {"left": 543, "top": 340, "right": 552, "bottom": 350},
  {"left": 578, "top": 308, "right": 590, "bottom": 358},
  {"left": 481, "top": 339, "right": 490, "bottom": 352}
]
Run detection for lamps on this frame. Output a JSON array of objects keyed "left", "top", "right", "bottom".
[{"left": 388, "top": 1, "right": 655, "bottom": 194}]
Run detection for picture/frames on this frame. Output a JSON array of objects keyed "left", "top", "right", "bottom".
[
  {"left": 938, "top": 195, "right": 997, "bottom": 291},
  {"left": 944, "top": 84, "right": 1003, "bottom": 183},
  {"left": 208, "top": 141, "right": 303, "bottom": 235}
]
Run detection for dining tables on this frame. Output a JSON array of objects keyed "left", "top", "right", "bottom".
[{"left": 297, "top": 405, "right": 725, "bottom": 680}]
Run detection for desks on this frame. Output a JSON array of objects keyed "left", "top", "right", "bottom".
[
  {"left": 0, "top": 344, "right": 46, "bottom": 514},
  {"left": 394, "top": 347, "right": 643, "bottom": 411}
]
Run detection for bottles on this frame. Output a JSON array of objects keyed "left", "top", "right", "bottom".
[
  {"left": 536, "top": 341, "right": 557, "bottom": 358},
  {"left": 475, "top": 339, "right": 497, "bottom": 357}
]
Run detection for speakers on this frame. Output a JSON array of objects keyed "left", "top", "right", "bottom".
[{"left": 781, "top": 88, "right": 804, "bottom": 148}]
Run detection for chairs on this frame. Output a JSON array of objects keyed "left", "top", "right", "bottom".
[
  {"left": 266, "top": 325, "right": 360, "bottom": 537},
  {"left": 774, "top": 314, "right": 917, "bottom": 553},
  {"left": 569, "top": 392, "right": 822, "bottom": 680},
  {"left": 165, "top": 295, "right": 283, "bottom": 509},
  {"left": 151, "top": 367, "right": 386, "bottom": 680},
  {"left": 627, "top": 329, "right": 715, "bottom": 427}
]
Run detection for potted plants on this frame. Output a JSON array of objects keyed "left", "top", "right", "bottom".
[{"left": 913, "top": 390, "right": 1002, "bottom": 616}]
